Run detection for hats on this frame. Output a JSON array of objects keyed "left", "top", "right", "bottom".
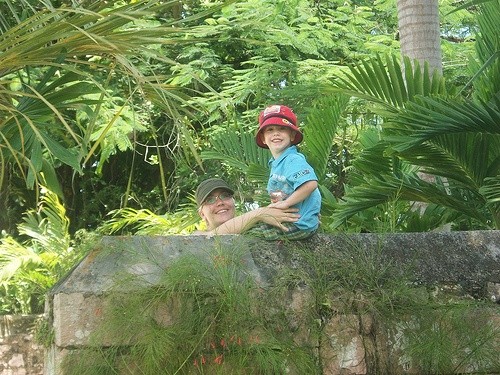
[
  {"left": 195, "top": 178, "right": 234, "bottom": 207},
  {"left": 256, "top": 105, "right": 303, "bottom": 149}
]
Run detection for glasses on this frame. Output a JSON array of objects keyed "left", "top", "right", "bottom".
[{"left": 202, "top": 192, "right": 232, "bottom": 206}]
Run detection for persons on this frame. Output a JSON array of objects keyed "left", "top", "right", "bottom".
[
  {"left": 190, "top": 178, "right": 322, "bottom": 236},
  {"left": 248, "top": 105, "right": 322, "bottom": 241}
]
